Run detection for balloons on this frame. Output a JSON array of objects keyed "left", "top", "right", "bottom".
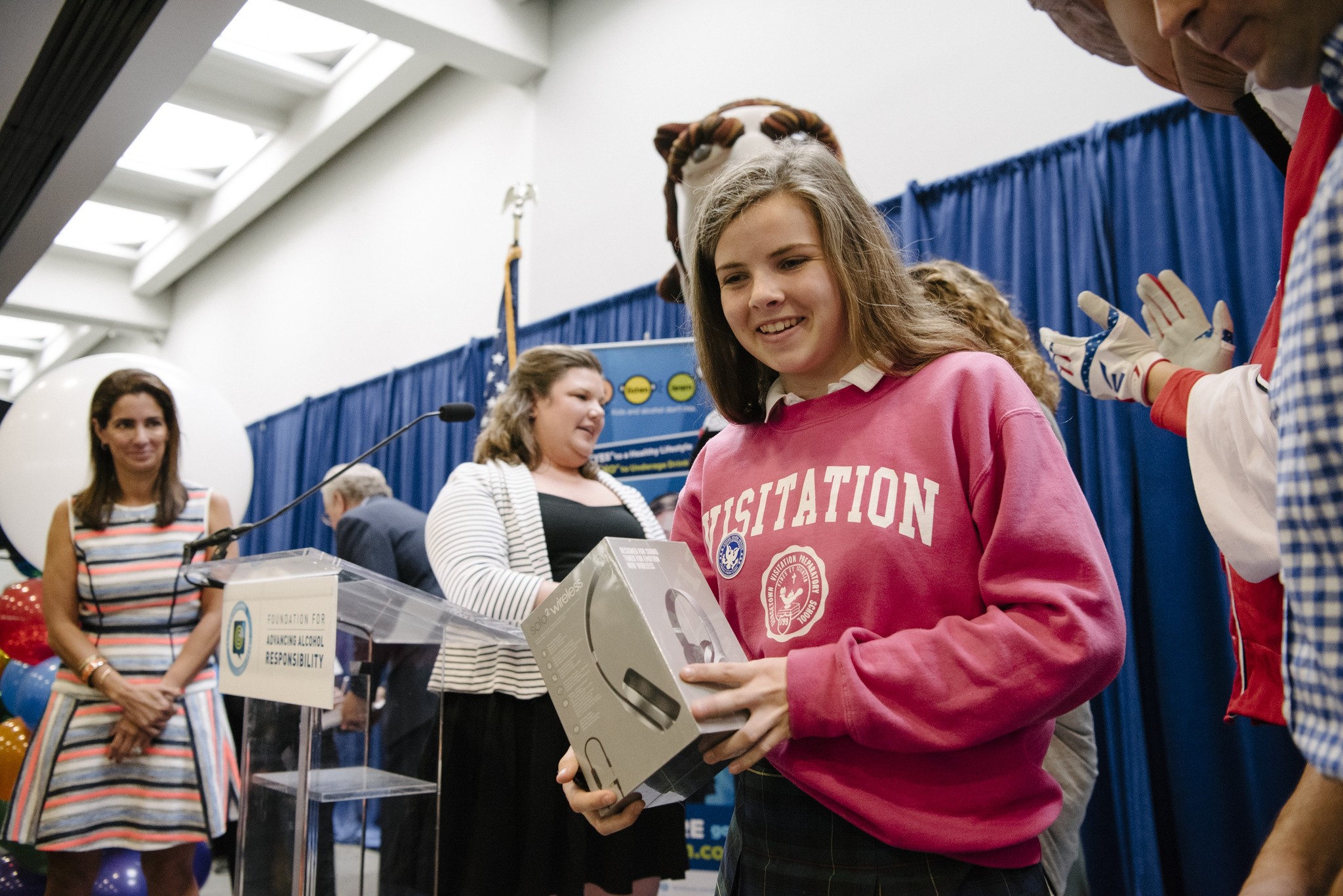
[{"left": 0, "top": 575, "right": 212, "bottom": 896}]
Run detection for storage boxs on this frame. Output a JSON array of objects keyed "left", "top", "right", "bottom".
[{"left": 520, "top": 536, "right": 753, "bottom": 819}]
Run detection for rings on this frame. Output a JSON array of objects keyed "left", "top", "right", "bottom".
[{"left": 130, "top": 747, "right": 142, "bottom": 754}]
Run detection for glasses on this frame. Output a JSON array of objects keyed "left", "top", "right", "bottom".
[{"left": 321, "top": 499, "right": 337, "bottom": 528}]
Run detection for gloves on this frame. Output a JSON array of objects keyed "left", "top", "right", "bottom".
[
  {"left": 1135, "top": 270, "right": 1236, "bottom": 375},
  {"left": 1039, "top": 292, "right": 1171, "bottom": 409}
]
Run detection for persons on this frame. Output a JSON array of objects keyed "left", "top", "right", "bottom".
[
  {"left": 321, "top": 463, "right": 449, "bottom": 896},
  {"left": 423, "top": 346, "right": 667, "bottom": 896},
  {"left": 209, "top": 693, "right": 336, "bottom": 896},
  {"left": 910, "top": 259, "right": 1097, "bottom": 896},
  {"left": 0, "top": 368, "right": 243, "bottom": 896},
  {"left": 556, "top": 138, "right": 1126, "bottom": 896},
  {"left": 1040, "top": 0, "right": 1343, "bottom": 896}
]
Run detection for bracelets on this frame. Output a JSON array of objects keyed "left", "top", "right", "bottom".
[{"left": 74, "top": 654, "right": 113, "bottom": 691}]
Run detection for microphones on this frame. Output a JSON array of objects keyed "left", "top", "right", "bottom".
[{"left": 179, "top": 402, "right": 476, "bottom": 550}]
